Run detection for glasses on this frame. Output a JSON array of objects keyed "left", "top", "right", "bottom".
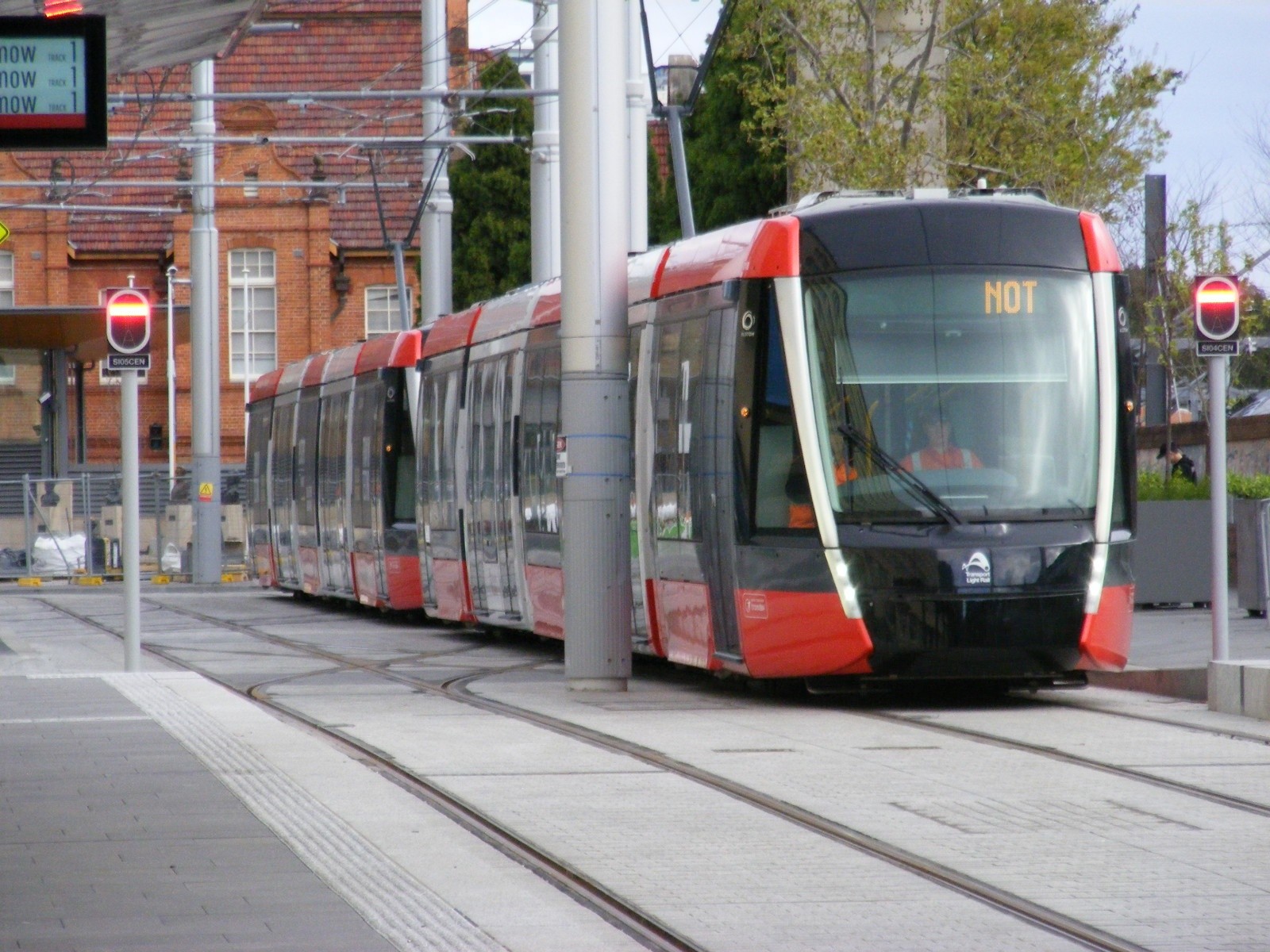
[{"left": 925, "top": 414, "right": 952, "bottom": 425}]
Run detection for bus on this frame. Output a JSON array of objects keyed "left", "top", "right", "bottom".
[{"left": 244, "top": 0, "right": 1176, "bottom": 691}]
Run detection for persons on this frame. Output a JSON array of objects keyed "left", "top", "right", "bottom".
[
  {"left": 788, "top": 416, "right": 857, "bottom": 529},
  {"left": 895, "top": 409, "right": 984, "bottom": 472},
  {"left": 1155, "top": 441, "right": 1197, "bottom": 487}
]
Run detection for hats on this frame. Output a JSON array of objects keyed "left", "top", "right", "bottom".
[{"left": 1157, "top": 442, "right": 1177, "bottom": 459}]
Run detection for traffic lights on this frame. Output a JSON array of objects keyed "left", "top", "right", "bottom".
[
  {"left": 106, "top": 289, "right": 152, "bottom": 354},
  {"left": 1193, "top": 273, "right": 1241, "bottom": 343}
]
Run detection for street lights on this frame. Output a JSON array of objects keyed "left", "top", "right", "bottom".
[{"left": 165, "top": 264, "right": 192, "bottom": 499}]
